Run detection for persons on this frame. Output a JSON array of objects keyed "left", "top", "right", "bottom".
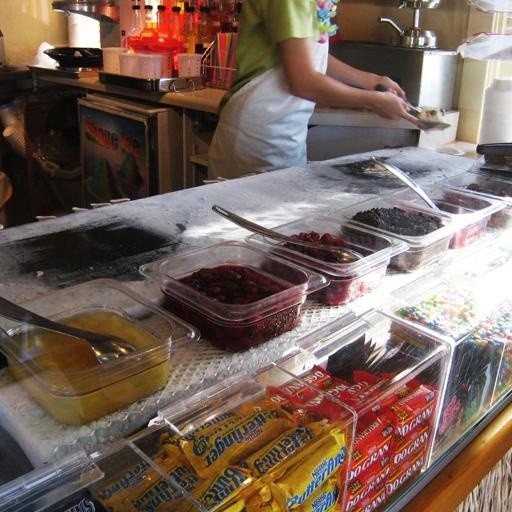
[{"left": 205, "top": 0, "right": 418, "bottom": 181}]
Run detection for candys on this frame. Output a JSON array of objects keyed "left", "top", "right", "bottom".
[{"left": 386, "top": 284, "right": 511, "bottom": 390}]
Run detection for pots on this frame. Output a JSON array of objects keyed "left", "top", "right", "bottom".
[{"left": 44, "top": 46, "right": 104, "bottom": 67}]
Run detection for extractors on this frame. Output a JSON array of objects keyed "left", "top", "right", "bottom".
[{"left": 51, "top": 0, "right": 120, "bottom": 25}]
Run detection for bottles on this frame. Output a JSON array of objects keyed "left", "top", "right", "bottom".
[
  {"left": 126, "top": 0, "right": 241, "bottom": 77},
  {"left": 120, "top": 31, "right": 126, "bottom": 47}
]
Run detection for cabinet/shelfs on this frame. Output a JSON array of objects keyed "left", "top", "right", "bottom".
[{"left": 182, "top": 109, "right": 220, "bottom": 189}]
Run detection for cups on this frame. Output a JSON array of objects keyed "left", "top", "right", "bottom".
[{"left": 101, "top": 47, "right": 202, "bottom": 78}]
